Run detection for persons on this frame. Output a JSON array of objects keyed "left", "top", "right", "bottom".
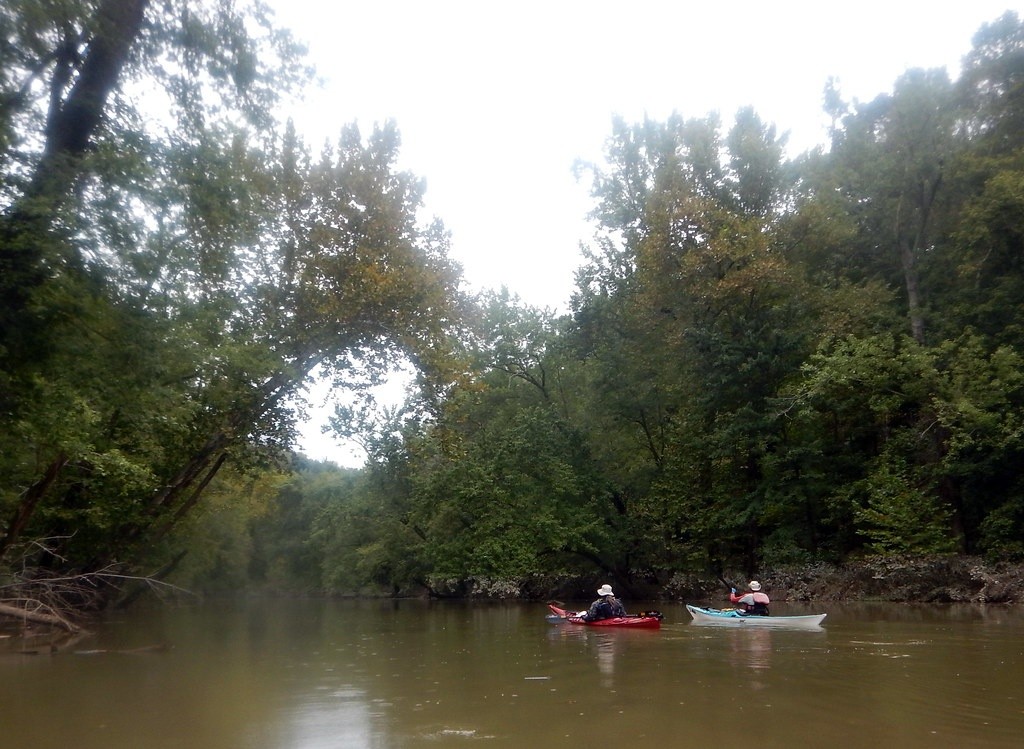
[
  {"left": 586, "top": 584, "right": 622, "bottom": 619},
  {"left": 730, "top": 581, "right": 769, "bottom": 616}
]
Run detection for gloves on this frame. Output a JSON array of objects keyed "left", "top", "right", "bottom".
[{"left": 732, "top": 588, "right": 736, "bottom": 593}]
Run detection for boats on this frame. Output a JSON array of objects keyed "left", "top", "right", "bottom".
[
  {"left": 686, "top": 605, "right": 827, "bottom": 626},
  {"left": 546, "top": 600, "right": 659, "bottom": 628}
]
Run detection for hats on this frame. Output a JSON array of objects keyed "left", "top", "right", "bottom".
[
  {"left": 597, "top": 584, "right": 614, "bottom": 596},
  {"left": 748, "top": 581, "right": 761, "bottom": 590}
]
